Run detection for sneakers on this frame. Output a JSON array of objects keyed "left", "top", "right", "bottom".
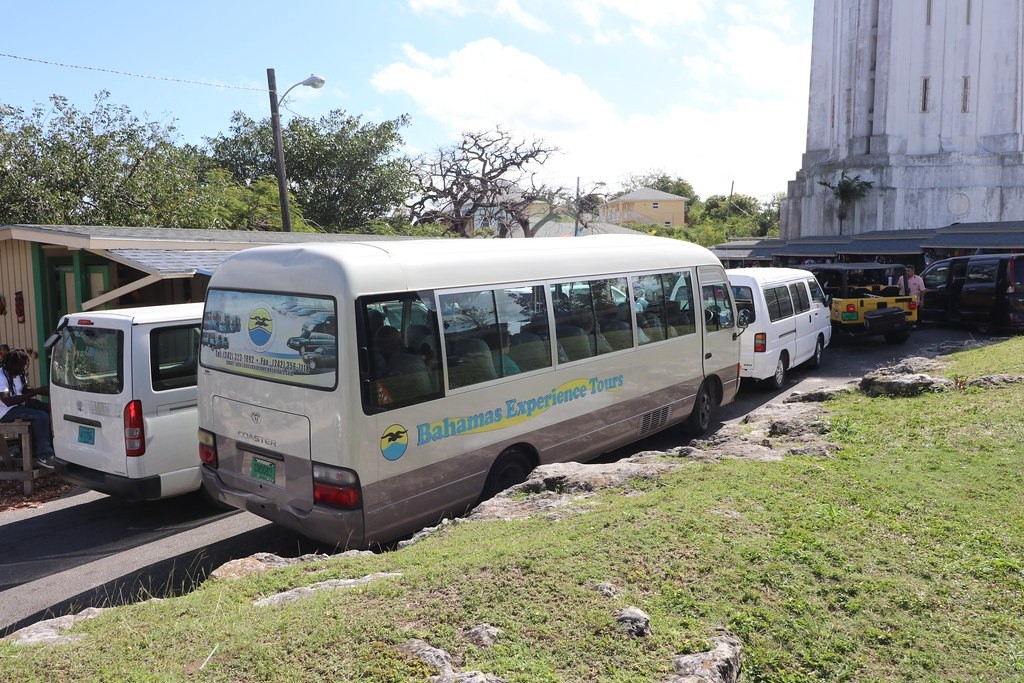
[{"left": 37, "top": 458, "right": 55, "bottom": 468}]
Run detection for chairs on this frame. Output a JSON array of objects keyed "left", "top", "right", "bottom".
[
  {"left": 376, "top": 299, "right": 690, "bottom": 395},
  {"left": 850, "top": 288, "right": 869, "bottom": 298},
  {"left": 880, "top": 285, "right": 901, "bottom": 297}
]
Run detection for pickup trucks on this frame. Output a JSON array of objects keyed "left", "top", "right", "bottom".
[{"left": 804, "top": 263, "right": 917, "bottom": 344}]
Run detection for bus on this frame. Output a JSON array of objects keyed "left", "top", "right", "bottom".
[{"left": 197, "top": 233, "right": 750, "bottom": 550}]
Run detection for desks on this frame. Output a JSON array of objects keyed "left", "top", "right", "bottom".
[{"left": 1, "top": 418, "right": 53, "bottom": 496}]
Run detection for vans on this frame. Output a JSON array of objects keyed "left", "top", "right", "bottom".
[
  {"left": 725, "top": 267, "right": 833, "bottom": 389},
  {"left": 43, "top": 302, "right": 237, "bottom": 512},
  {"left": 918, "top": 253, "right": 1024, "bottom": 336}
]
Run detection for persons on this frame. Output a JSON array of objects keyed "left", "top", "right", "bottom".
[
  {"left": 366, "top": 284, "right": 650, "bottom": 399},
  {"left": 897, "top": 265, "right": 925, "bottom": 335},
  {"left": 0, "top": 343, "right": 55, "bottom": 468}
]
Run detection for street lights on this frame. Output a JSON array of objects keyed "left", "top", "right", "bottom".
[
  {"left": 573, "top": 176, "right": 606, "bottom": 236},
  {"left": 266, "top": 68, "right": 326, "bottom": 232}
]
